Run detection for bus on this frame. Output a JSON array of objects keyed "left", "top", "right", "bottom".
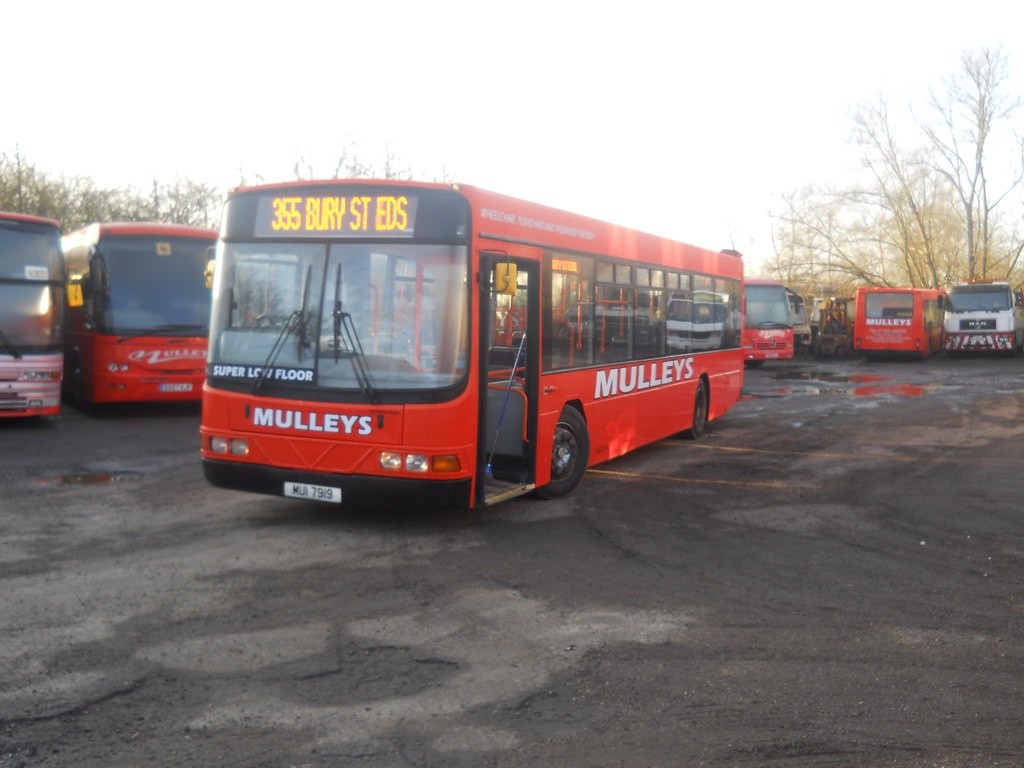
[
  {"left": 667, "top": 289, "right": 731, "bottom": 352},
  {"left": 59, "top": 221, "right": 224, "bottom": 412},
  {"left": 739, "top": 281, "right": 803, "bottom": 372},
  {"left": 0, "top": 210, "right": 84, "bottom": 422},
  {"left": 942, "top": 281, "right": 1023, "bottom": 355},
  {"left": 787, "top": 292, "right": 812, "bottom": 352},
  {"left": 198, "top": 178, "right": 746, "bottom": 513},
  {"left": 854, "top": 286, "right": 948, "bottom": 359}
]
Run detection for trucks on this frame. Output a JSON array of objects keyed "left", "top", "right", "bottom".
[{"left": 597, "top": 282, "right": 664, "bottom": 348}]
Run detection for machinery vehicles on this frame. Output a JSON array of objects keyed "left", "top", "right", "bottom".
[{"left": 807, "top": 295, "right": 855, "bottom": 359}]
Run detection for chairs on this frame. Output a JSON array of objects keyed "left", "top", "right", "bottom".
[{"left": 488, "top": 346, "right": 525, "bottom": 378}]
